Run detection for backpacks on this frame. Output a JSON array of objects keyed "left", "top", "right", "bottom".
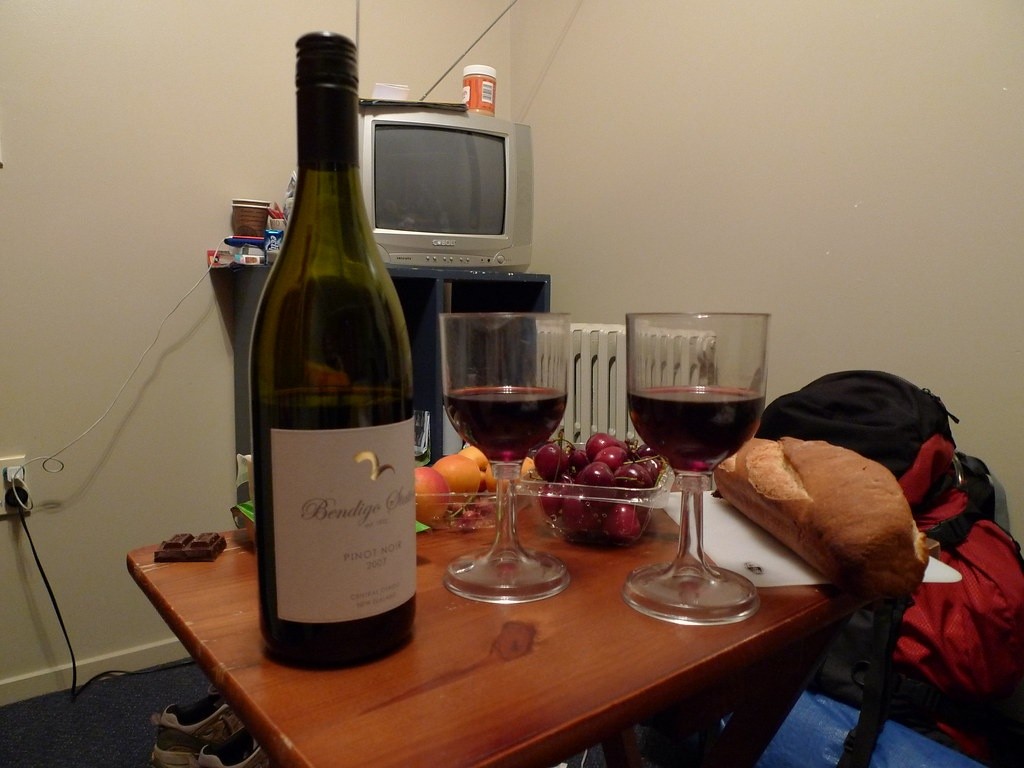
[{"left": 821, "top": 495, "right": 1024, "bottom": 768}]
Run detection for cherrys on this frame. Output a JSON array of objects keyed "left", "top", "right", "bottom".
[{"left": 535, "top": 429, "right": 670, "bottom": 545}]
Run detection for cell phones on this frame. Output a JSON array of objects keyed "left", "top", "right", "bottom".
[{"left": 233, "top": 235, "right": 265, "bottom": 240}]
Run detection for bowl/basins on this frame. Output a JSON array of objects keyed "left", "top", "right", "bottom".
[
  {"left": 520, "top": 443, "right": 675, "bottom": 548},
  {"left": 415, "top": 491, "right": 527, "bottom": 530}
]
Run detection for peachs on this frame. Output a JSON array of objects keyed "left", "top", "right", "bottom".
[{"left": 413, "top": 446, "right": 535, "bottom": 526}]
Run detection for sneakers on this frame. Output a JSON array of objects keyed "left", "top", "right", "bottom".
[{"left": 152, "top": 686, "right": 268, "bottom": 768}]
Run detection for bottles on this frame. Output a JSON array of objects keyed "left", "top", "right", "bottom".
[
  {"left": 248, "top": 31, "right": 415, "bottom": 670},
  {"left": 463, "top": 65, "right": 497, "bottom": 115}
]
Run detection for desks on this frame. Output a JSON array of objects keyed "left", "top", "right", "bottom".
[{"left": 126, "top": 474, "right": 874, "bottom": 768}]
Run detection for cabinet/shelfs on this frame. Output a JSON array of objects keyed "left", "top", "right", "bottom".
[{"left": 206, "top": 266, "right": 551, "bottom": 504}]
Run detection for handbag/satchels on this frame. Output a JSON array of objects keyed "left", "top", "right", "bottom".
[
  {"left": 747, "top": 367, "right": 968, "bottom": 519},
  {"left": 756, "top": 693, "right": 987, "bottom": 768}
]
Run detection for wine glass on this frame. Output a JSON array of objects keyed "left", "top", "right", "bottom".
[
  {"left": 624, "top": 311, "right": 769, "bottom": 627},
  {"left": 437, "top": 316, "right": 573, "bottom": 604}
]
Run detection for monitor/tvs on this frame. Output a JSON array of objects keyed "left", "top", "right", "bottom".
[{"left": 358, "top": 105, "right": 534, "bottom": 267}]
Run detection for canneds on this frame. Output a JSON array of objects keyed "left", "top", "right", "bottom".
[{"left": 462, "top": 65, "right": 496, "bottom": 117}]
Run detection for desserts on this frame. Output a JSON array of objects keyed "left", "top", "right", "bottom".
[{"left": 153, "top": 532, "right": 224, "bottom": 562}]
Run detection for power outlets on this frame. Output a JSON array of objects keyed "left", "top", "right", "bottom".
[{"left": 0, "top": 456, "right": 31, "bottom": 515}]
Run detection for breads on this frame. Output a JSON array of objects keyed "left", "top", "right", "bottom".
[{"left": 715, "top": 436, "right": 929, "bottom": 603}]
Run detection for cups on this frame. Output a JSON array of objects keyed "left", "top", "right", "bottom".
[{"left": 232, "top": 199, "right": 270, "bottom": 236}]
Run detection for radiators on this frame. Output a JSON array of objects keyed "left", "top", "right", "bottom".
[{"left": 535, "top": 320, "right": 714, "bottom": 451}]
